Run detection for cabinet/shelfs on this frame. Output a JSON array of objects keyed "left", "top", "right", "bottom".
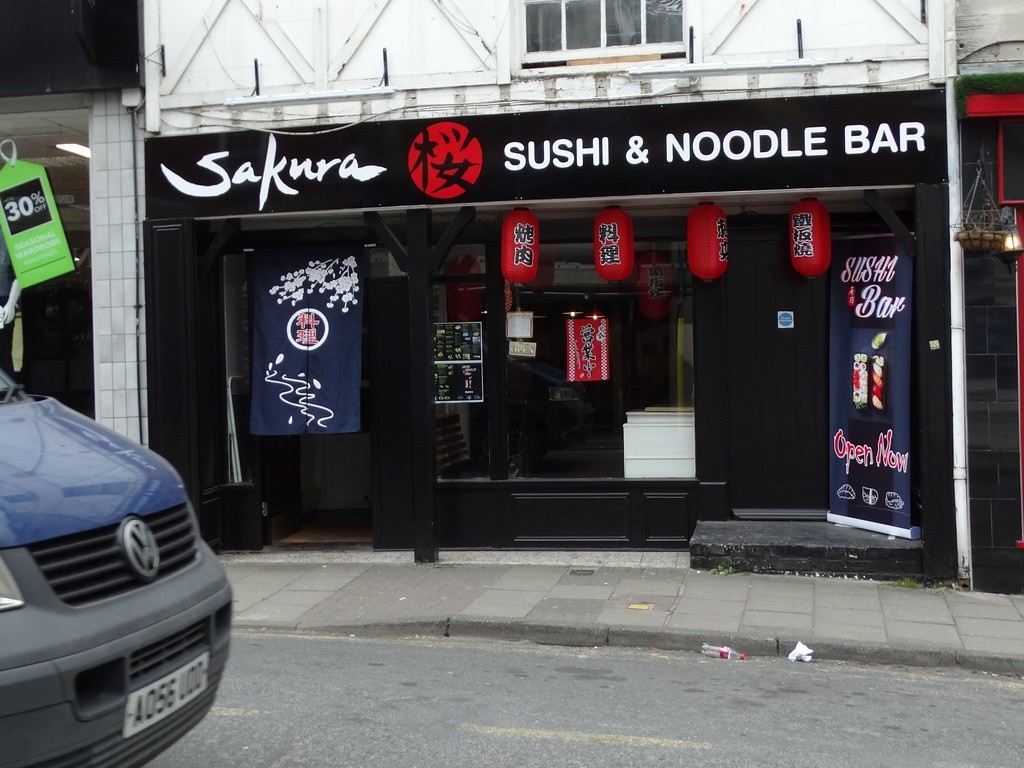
[
  {"left": 622, "top": 401, "right": 696, "bottom": 482},
  {"left": 299, "top": 433, "right": 372, "bottom": 509}
]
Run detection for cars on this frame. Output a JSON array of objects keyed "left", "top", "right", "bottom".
[
  {"left": 507, "top": 361, "right": 599, "bottom": 462},
  {"left": 0, "top": 369, "right": 234, "bottom": 768}
]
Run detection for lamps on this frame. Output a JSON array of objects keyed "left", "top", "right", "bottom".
[
  {"left": 993, "top": 215, "right": 1024, "bottom": 274},
  {"left": 561, "top": 303, "right": 606, "bottom": 320}
]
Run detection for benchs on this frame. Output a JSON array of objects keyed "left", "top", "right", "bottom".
[{"left": 434, "top": 413, "right": 519, "bottom": 480}]
